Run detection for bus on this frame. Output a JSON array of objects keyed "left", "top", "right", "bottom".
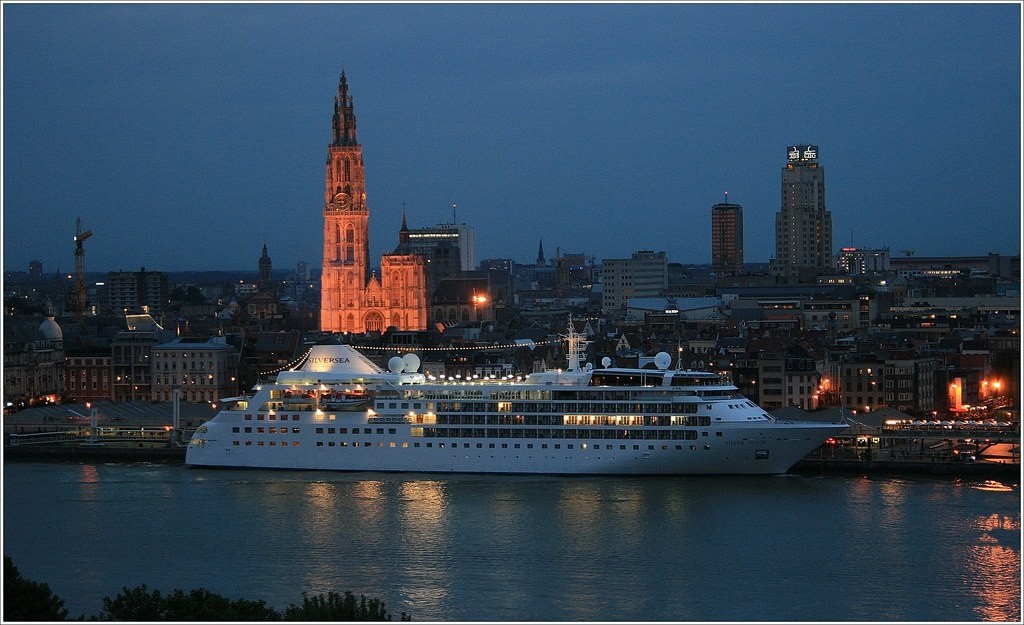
[
  {"left": 959, "top": 445, "right": 976, "bottom": 462},
  {"left": 924, "top": 441, "right": 949, "bottom": 451}
]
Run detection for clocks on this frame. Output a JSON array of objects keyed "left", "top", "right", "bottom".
[{"left": 333, "top": 193, "right": 351, "bottom": 211}]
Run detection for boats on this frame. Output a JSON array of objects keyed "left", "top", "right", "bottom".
[{"left": 185, "top": 314, "right": 849, "bottom": 478}]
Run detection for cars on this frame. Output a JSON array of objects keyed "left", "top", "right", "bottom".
[
  {"left": 973, "top": 438, "right": 989, "bottom": 444},
  {"left": 958, "top": 439, "right": 971, "bottom": 443}
]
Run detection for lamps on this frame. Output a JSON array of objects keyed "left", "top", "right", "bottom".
[{"left": 428, "top": 373, "right": 523, "bottom": 381}]
